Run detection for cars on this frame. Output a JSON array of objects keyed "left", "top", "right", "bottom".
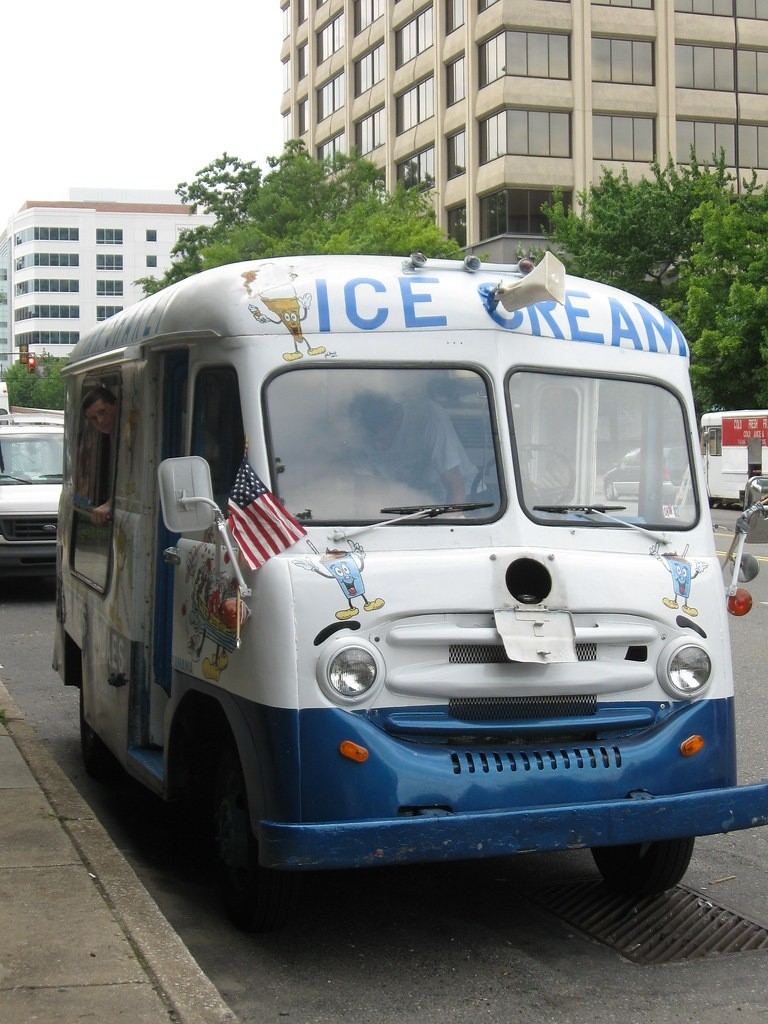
[
  {"left": 1, "top": 407, "right": 63, "bottom": 596},
  {"left": 603, "top": 447, "right": 686, "bottom": 500}
]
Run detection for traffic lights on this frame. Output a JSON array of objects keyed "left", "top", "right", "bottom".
[{"left": 28, "top": 357, "right": 36, "bottom": 374}]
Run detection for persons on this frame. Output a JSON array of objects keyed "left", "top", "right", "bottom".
[
  {"left": 81, "top": 387, "right": 118, "bottom": 526},
  {"left": 348, "top": 391, "right": 487, "bottom": 519}
]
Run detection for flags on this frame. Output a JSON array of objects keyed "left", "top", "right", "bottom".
[{"left": 226, "top": 459, "right": 307, "bottom": 571}]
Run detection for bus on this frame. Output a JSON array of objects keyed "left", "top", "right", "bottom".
[
  {"left": 700, "top": 409, "right": 768, "bottom": 508},
  {"left": 52, "top": 253, "right": 768, "bottom": 932}
]
